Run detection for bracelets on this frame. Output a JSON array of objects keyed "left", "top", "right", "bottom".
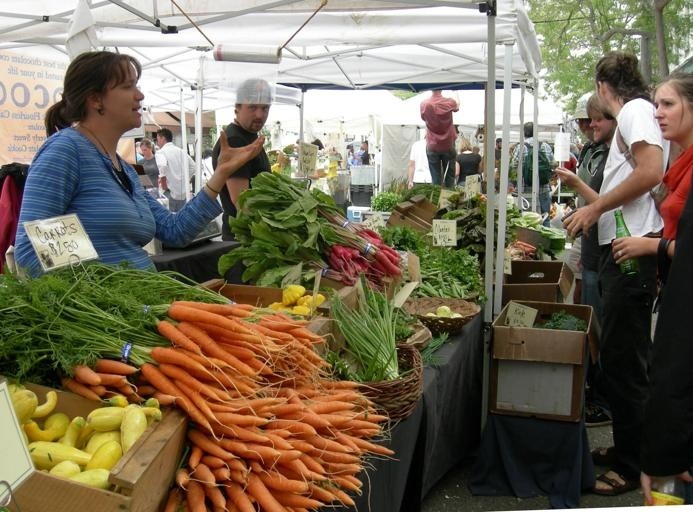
[{"left": 205, "top": 182, "right": 220, "bottom": 194}]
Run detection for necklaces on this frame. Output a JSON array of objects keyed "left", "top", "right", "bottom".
[{"left": 78, "top": 124, "right": 123, "bottom": 173}]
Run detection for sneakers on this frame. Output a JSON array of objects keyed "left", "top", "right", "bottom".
[{"left": 585, "top": 405, "right": 613, "bottom": 427}]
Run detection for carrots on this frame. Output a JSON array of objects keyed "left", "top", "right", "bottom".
[{"left": 62, "top": 302, "right": 401, "bottom": 512}]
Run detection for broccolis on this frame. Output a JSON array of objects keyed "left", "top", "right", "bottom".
[{"left": 543, "top": 309, "right": 587, "bottom": 331}]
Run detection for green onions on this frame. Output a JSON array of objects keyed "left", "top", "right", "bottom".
[{"left": 326, "top": 271, "right": 413, "bottom": 384}]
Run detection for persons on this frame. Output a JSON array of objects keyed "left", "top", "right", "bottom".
[
  {"left": 420, "top": 90, "right": 583, "bottom": 229},
  {"left": 132, "top": 127, "right": 215, "bottom": 212},
  {"left": 311, "top": 142, "right": 369, "bottom": 207},
  {"left": 13, "top": 50, "right": 267, "bottom": 278},
  {"left": 553, "top": 50, "right": 693, "bottom": 505},
  {"left": 210, "top": 78, "right": 271, "bottom": 238}
]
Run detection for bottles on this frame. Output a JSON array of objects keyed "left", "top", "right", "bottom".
[{"left": 614, "top": 209, "right": 640, "bottom": 277}]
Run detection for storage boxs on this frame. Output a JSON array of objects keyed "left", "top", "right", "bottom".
[{"left": 492, "top": 259, "right": 602, "bottom": 424}]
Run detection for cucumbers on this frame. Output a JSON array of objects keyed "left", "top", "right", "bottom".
[{"left": 8, "top": 381, "right": 163, "bottom": 490}]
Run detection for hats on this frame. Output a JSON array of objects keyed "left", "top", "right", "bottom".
[
  {"left": 569, "top": 90, "right": 599, "bottom": 119},
  {"left": 235, "top": 78, "right": 271, "bottom": 106}
]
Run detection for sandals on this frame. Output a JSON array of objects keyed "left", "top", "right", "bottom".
[
  {"left": 591, "top": 446, "right": 615, "bottom": 463},
  {"left": 594, "top": 469, "right": 641, "bottom": 496}
]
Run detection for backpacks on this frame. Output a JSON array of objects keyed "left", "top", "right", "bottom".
[{"left": 521, "top": 137, "right": 553, "bottom": 186}]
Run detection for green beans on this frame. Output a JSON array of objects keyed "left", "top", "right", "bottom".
[{"left": 394, "top": 263, "right": 474, "bottom": 303}]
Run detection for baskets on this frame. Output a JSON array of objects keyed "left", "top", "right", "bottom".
[
  {"left": 403, "top": 295, "right": 481, "bottom": 334},
  {"left": 340, "top": 342, "right": 424, "bottom": 421}
]
